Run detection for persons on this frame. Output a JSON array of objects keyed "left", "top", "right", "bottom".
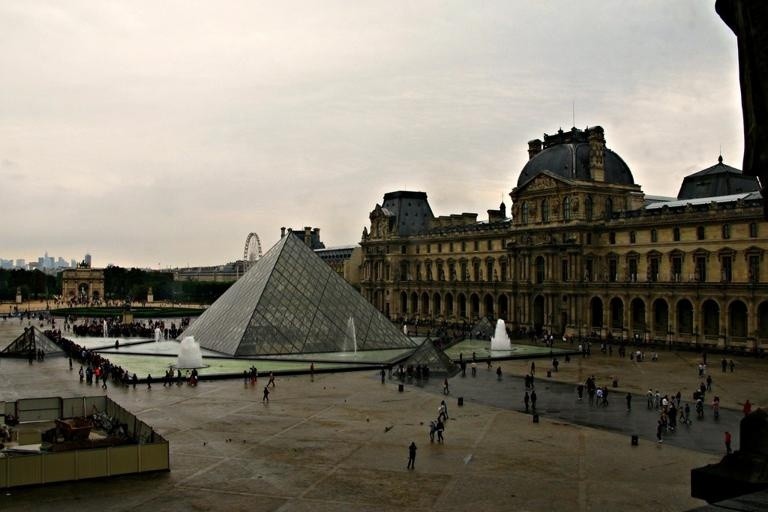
[
  {"left": 386, "top": 314, "right": 495, "bottom": 340},
  {"left": 379, "top": 362, "right": 450, "bottom": 395},
  {"left": 625, "top": 375, "right": 757, "bottom": 455},
  {"left": 0, "top": 293, "right": 219, "bottom": 393},
  {"left": 697, "top": 348, "right": 736, "bottom": 376},
  {"left": 523, "top": 359, "right": 559, "bottom": 412},
  {"left": 562, "top": 335, "right": 658, "bottom": 363},
  {"left": 460, "top": 352, "right": 502, "bottom": 379},
  {"left": 585, "top": 373, "right": 618, "bottom": 410},
  {"left": 429, "top": 401, "right": 448, "bottom": 443},
  {"left": 401, "top": 439, "right": 419, "bottom": 472},
  {"left": 305, "top": 361, "right": 318, "bottom": 385},
  {"left": 506, "top": 326, "right": 553, "bottom": 347},
  {"left": 241, "top": 366, "right": 282, "bottom": 401}
]
